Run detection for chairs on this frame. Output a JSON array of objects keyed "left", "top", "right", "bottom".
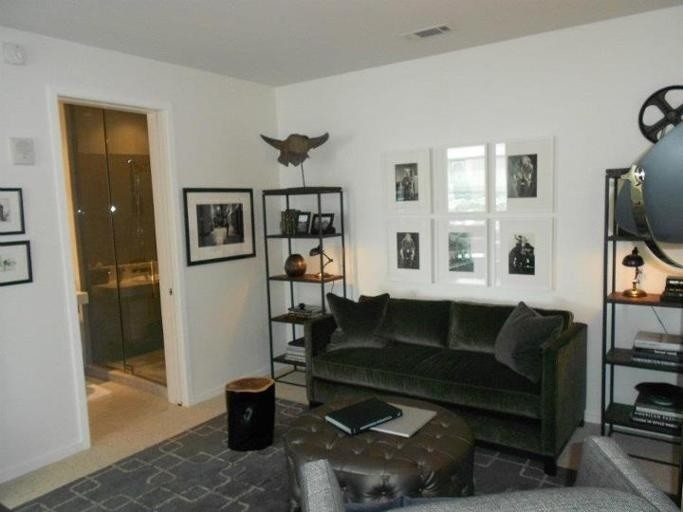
[{"left": 295, "top": 435, "right": 679, "bottom": 511}]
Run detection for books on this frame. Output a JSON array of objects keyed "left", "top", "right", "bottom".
[
  {"left": 368, "top": 401, "right": 437, "bottom": 438},
  {"left": 631, "top": 381, "right": 682, "bottom": 435},
  {"left": 628, "top": 330, "right": 683, "bottom": 373},
  {"left": 283, "top": 303, "right": 323, "bottom": 363},
  {"left": 323, "top": 396, "right": 403, "bottom": 436},
  {"left": 659, "top": 275, "right": 683, "bottom": 302}
]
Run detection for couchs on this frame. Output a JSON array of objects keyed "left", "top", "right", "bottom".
[{"left": 305, "top": 300, "right": 588, "bottom": 478}]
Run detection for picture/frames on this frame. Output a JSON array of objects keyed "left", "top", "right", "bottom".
[
  {"left": 0, "top": 187, "right": 33, "bottom": 286},
  {"left": 182, "top": 187, "right": 257, "bottom": 267},
  {"left": 294, "top": 211, "right": 335, "bottom": 234},
  {"left": 383, "top": 133, "right": 556, "bottom": 290}
]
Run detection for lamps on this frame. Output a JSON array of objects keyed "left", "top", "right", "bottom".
[
  {"left": 622, "top": 246, "right": 648, "bottom": 298},
  {"left": 309, "top": 244, "right": 334, "bottom": 277}
]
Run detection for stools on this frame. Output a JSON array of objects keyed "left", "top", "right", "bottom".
[{"left": 281, "top": 392, "right": 476, "bottom": 509}]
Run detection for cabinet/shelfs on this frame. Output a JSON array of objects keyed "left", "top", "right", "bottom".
[
  {"left": 600, "top": 166, "right": 683, "bottom": 467},
  {"left": 261, "top": 186, "right": 346, "bottom": 389}
]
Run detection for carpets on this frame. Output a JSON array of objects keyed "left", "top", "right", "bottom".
[{"left": 0, "top": 397, "right": 679, "bottom": 512}]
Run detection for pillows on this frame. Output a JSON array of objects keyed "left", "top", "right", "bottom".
[
  {"left": 322, "top": 291, "right": 389, "bottom": 353},
  {"left": 492, "top": 300, "right": 563, "bottom": 385}
]
